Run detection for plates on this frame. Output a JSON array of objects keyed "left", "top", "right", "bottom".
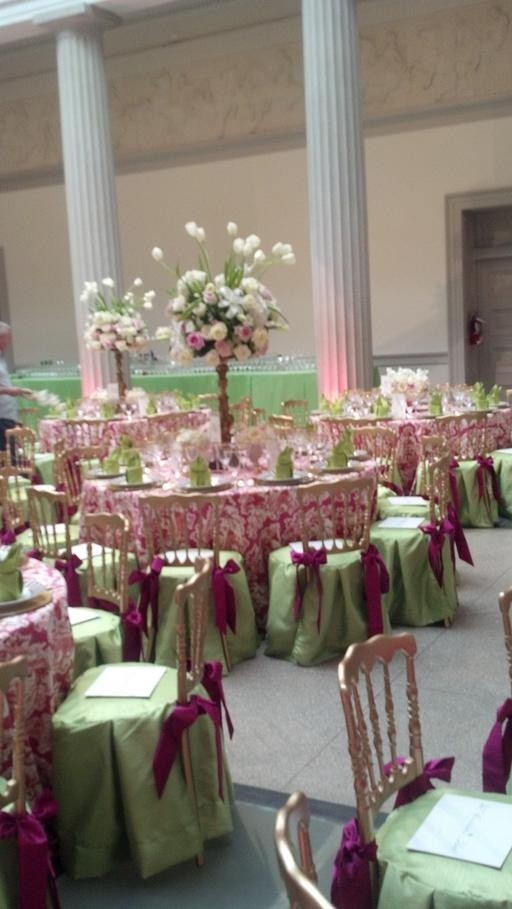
[
  {"left": 0, "top": 576, "right": 46, "bottom": 609},
  {"left": 92, "top": 449, "right": 371, "bottom": 491}
]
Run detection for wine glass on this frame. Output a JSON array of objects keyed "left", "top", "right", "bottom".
[{"left": 147, "top": 382, "right": 477, "bottom": 474}]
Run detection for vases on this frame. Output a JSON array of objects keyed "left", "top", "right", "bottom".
[{"left": 115, "top": 349, "right": 126, "bottom": 413}]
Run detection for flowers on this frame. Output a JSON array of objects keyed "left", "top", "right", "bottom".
[
  {"left": 78, "top": 277, "right": 155, "bottom": 355},
  {"left": 152, "top": 220, "right": 297, "bottom": 368}
]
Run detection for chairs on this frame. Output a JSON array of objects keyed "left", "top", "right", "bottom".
[
  {"left": 337, "top": 632, "right": 512, "bottom": 909},
  {"left": 275, "top": 793, "right": 337, "bottom": 909},
  {"left": 0, "top": 657, "right": 62, "bottom": 909},
  {"left": 483, "top": 589, "right": 512, "bottom": 796},
  {"left": 53, "top": 557, "right": 233, "bottom": 879}
]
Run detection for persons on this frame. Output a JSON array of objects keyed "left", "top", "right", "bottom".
[{"left": 0, "top": 320, "right": 37, "bottom": 456}]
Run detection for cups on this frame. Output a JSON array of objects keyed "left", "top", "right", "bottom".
[{"left": 26, "top": 353, "right": 316, "bottom": 378}]
